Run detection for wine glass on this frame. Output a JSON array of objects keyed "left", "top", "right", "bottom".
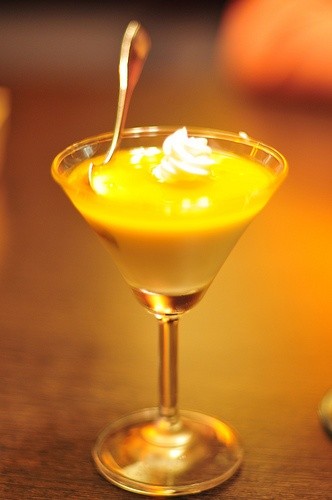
[{"left": 50, "top": 125, "right": 288, "bottom": 494}]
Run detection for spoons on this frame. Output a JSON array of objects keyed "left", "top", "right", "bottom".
[{"left": 86, "top": 21, "right": 149, "bottom": 193}]
